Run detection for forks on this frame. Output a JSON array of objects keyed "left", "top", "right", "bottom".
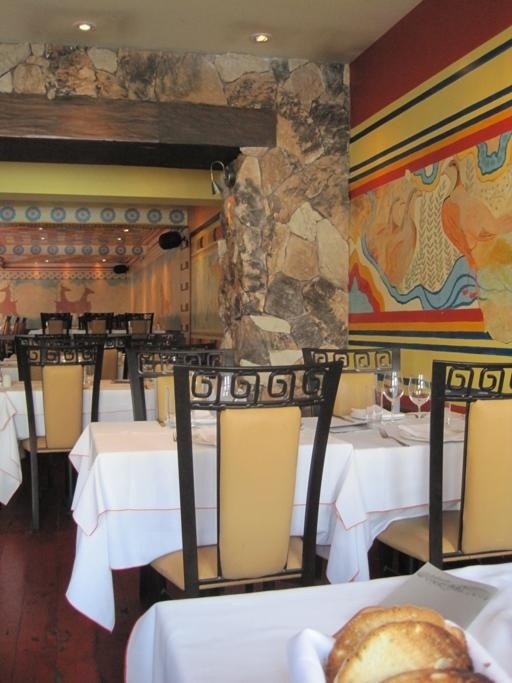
[{"left": 380, "top": 429, "right": 409, "bottom": 448}]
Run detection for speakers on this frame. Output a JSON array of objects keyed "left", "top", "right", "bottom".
[
  {"left": 113, "top": 265, "right": 128, "bottom": 274},
  {"left": 159, "top": 231, "right": 182, "bottom": 249}
]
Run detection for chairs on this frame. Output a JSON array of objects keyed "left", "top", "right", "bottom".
[
  {"left": 141, "top": 363, "right": 343, "bottom": 595},
  {"left": 1, "top": 313, "right": 217, "bottom": 506},
  {"left": 301, "top": 345, "right": 401, "bottom": 413},
  {"left": 126, "top": 349, "right": 218, "bottom": 421},
  {"left": 14, "top": 335, "right": 105, "bottom": 531},
  {"left": 376, "top": 359, "right": 512, "bottom": 572}
]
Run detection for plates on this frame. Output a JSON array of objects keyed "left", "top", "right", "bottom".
[
  {"left": 192, "top": 425, "right": 217, "bottom": 446},
  {"left": 351, "top": 409, "right": 404, "bottom": 421}
]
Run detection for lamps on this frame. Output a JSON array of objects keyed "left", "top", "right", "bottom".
[{"left": 210, "top": 160, "right": 235, "bottom": 195}]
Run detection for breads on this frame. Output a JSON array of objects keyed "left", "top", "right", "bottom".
[{"left": 326, "top": 604, "right": 494, "bottom": 683}]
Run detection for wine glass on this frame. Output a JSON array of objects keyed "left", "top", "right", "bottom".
[
  {"left": 382, "top": 369, "right": 404, "bottom": 422},
  {"left": 408, "top": 375, "right": 430, "bottom": 425}
]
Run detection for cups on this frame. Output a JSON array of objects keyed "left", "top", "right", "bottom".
[{"left": 201, "top": 372, "right": 238, "bottom": 417}]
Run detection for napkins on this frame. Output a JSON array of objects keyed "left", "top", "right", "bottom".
[
  {"left": 173, "top": 408, "right": 217, "bottom": 446},
  {"left": 351, "top": 404, "right": 405, "bottom": 421}
]
